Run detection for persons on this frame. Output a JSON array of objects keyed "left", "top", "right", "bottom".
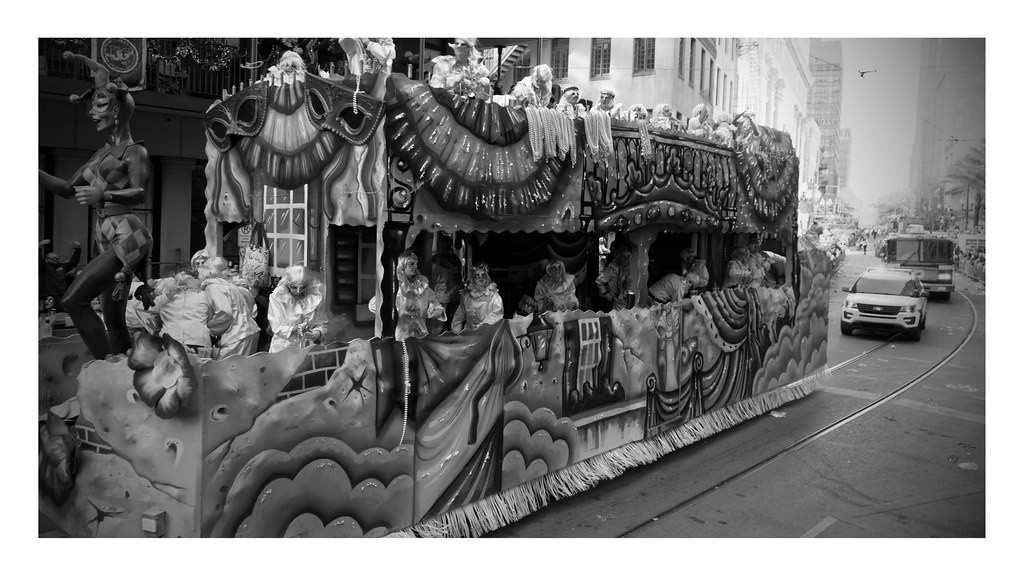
[
  {"left": 595, "top": 239, "right": 771, "bottom": 310},
  {"left": 124, "top": 250, "right": 281, "bottom": 360},
  {"left": 535, "top": 259, "right": 580, "bottom": 315},
  {"left": 855, "top": 228, "right": 878, "bottom": 256},
  {"left": 90, "top": 296, "right": 103, "bottom": 312},
  {"left": 267, "top": 265, "right": 329, "bottom": 354},
  {"left": 451, "top": 265, "right": 505, "bottom": 334},
  {"left": 368, "top": 248, "right": 449, "bottom": 342},
  {"left": 265, "top": 38, "right": 739, "bottom": 149},
  {"left": 38, "top": 238, "right": 82, "bottom": 300},
  {"left": 431, "top": 251, "right": 456, "bottom": 316},
  {"left": 38, "top": 84, "right": 154, "bottom": 361},
  {"left": 40, "top": 295, "right": 59, "bottom": 318}
]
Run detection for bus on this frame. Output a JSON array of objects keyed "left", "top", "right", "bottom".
[{"left": 880, "top": 233, "right": 957, "bottom": 299}]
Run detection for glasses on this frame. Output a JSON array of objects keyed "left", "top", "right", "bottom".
[
  {"left": 45, "top": 299, "right": 54, "bottom": 303},
  {"left": 48, "top": 256, "right": 60, "bottom": 260}
]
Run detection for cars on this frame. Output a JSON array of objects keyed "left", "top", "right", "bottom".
[{"left": 840, "top": 266, "right": 927, "bottom": 341}]
